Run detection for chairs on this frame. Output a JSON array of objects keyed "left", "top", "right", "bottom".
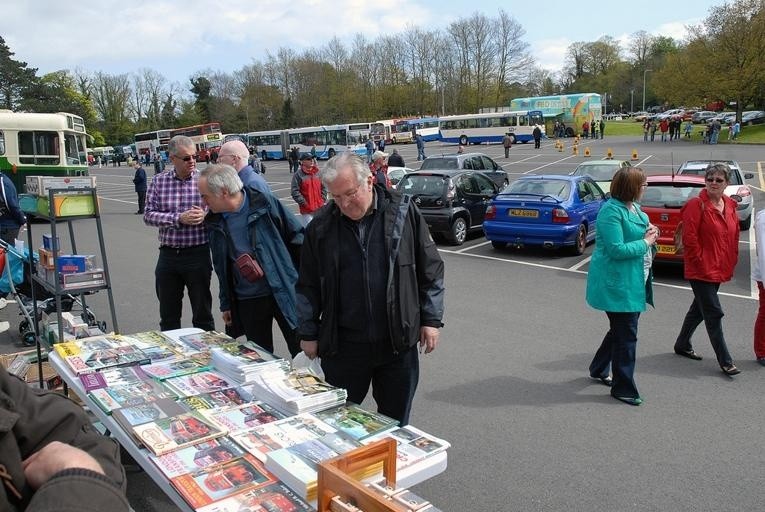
[{"left": 642, "top": 188, "right": 662, "bottom": 201}]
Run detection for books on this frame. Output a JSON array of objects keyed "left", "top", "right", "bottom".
[{"left": 52, "top": 321, "right": 450, "bottom": 511}]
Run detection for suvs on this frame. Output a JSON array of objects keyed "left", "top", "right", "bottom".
[{"left": 396, "top": 168, "right": 506, "bottom": 247}]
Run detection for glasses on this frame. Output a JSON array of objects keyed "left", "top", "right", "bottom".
[
  {"left": 707, "top": 178, "right": 723, "bottom": 182},
  {"left": 176, "top": 155, "right": 199, "bottom": 161}
]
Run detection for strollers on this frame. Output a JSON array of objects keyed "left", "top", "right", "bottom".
[{"left": 0, "top": 237, "right": 107, "bottom": 346}]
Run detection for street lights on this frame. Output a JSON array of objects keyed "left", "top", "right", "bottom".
[{"left": 642, "top": 67, "right": 653, "bottom": 103}]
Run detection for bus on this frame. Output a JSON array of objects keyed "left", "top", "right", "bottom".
[
  {"left": 0, "top": 108, "right": 96, "bottom": 223},
  {"left": 132, "top": 121, "right": 246, "bottom": 162},
  {"left": 437, "top": 110, "right": 546, "bottom": 144},
  {"left": 395, "top": 117, "right": 442, "bottom": 145},
  {"left": 244, "top": 129, "right": 289, "bottom": 160},
  {"left": 371, "top": 119, "right": 395, "bottom": 145},
  {"left": 507, "top": 94, "right": 603, "bottom": 140},
  {"left": 285, "top": 123, "right": 373, "bottom": 163}
]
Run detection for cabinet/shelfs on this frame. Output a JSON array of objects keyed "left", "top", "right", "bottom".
[{"left": 24, "top": 187, "right": 120, "bottom": 398}]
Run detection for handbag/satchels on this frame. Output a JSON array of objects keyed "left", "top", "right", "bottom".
[
  {"left": 674, "top": 220, "right": 687, "bottom": 254},
  {"left": 236, "top": 254, "right": 264, "bottom": 282}
]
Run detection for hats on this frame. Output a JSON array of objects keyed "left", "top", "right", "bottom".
[
  {"left": 372, "top": 151, "right": 390, "bottom": 160},
  {"left": 300, "top": 153, "right": 315, "bottom": 160}
]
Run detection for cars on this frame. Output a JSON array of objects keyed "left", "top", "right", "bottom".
[
  {"left": 642, "top": 174, "right": 713, "bottom": 262},
  {"left": 568, "top": 161, "right": 636, "bottom": 195},
  {"left": 420, "top": 154, "right": 510, "bottom": 192},
  {"left": 481, "top": 173, "right": 611, "bottom": 255},
  {"left": 675, "top": 159, "right": 753, "bottom": 229},
  {"left": 636, "top": 101, "right": 765, "bottom": 127},
  {"left": 383, "top": 166, "right": 418, "bottom": 190}
]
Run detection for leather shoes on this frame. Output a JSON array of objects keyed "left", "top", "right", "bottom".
[
  {"left": 676, "top": 348, "right": 703, "bottom": 360},
  {"left": 722, "top": 364, "right": 740, "bottom": 375},
  {"left": 588, "top": 373, "right": 642, "bottom": 405}
]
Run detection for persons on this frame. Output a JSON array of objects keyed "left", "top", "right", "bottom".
[
  {"left": 0, "top": 171, "right": 29, "bottom": 298},
  {"left": 365, "top": 136, "right": 405, "bottom": 175},
  {"left": 145, "top": 133, "right": 219, "bottom": 333},
  {"left": 643, "top": 116, "right": 691, "bottom": 142},
  {"left": 707, "top": 119, "right": 740, "bottom": 144},
  {"left": 132, "top": 163, "right": 147, "bottom": 214},
  {"left": 289, "top": 144, "right": 316, "bottom": 173},
  {"left": 458, "top": 146, "right": 464, "bottom": 153},
  {"left": 671, "top": 161, "right": 742, "bottom": 377},
  {"left": 582, "top": 120, "right": 604, "bottom": 139},
  {"left": 193, "top": 162, "right": 310, "bottom": 359},
  {"left": 1, "top": 361, "right": 139, "bottom": 511},
  {"left": 86, "top": 149, "right": 169, "bottom": 174},
  {"left": 583, "top": 161, "right": 661, "bottom": 409},
  {"left": 248, "top": 145, "right": 262, "bottom": 171},
  {"left": 623, "top": 109, "right": 627, "bottom": 114},
  {"left": 502, "top": 134, "right": 511, "bottom": 158},
  {"left": 205, "top": 148, "right": 218, "bottom": 163},
  {"left": 752, "top": 204, "right": 765, "bottom": 370},
  {"left": 416, "top": 134, "right": 427, "bottom": 161},
  {"left": 533, "top": 127, "right": 542, "bottom": 149},
  {"left": 555, "top": 122, "right": 565, "bottom": 137},
  {"left": 295, "top": 152, "right": 444, "bottom": 429}
]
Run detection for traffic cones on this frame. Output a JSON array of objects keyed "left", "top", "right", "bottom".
[{"left": 555, "top": 134, "right": 638, "bottom": 161}]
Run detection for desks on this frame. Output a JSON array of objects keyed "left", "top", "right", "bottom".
[{"left": 47, "top": 329, "right": 448, "bottom": 512}]
